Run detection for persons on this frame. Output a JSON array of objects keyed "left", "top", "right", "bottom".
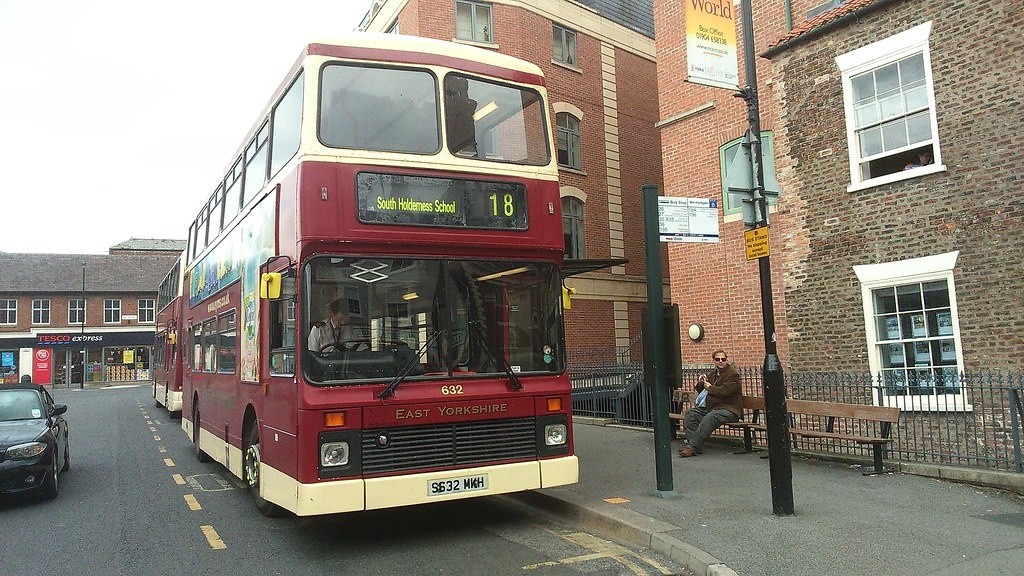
[
  {"left": 678, "top": 349, "right": 743, "bottom": 458},
  {"left": 309, "top": 298, "right": 369, "bottom": 353}
]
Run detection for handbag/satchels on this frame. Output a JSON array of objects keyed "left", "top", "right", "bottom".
[{"left": 695, "top": 378, "right": 716, "bottom": 408}]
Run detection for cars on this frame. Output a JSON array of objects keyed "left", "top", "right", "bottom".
[{"left": 0, "top": 383, "right": 70, "bottom": 501}]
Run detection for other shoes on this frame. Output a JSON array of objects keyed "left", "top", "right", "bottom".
[
  {"left": 679, "top": 448, "right": 697, "bottom": 457},
  {"left": 678, "top": 447, "right": 688, "bottom": 452}
]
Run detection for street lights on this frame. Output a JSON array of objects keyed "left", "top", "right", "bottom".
[{"left": 79, "top": 262, "right": 88, "bottom": 390}]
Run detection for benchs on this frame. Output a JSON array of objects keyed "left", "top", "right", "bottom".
[{"left": 669, "top": 390, "right": 901, "bottom": 476}]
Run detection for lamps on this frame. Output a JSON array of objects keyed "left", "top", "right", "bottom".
[{"left": 688, "top": 323, "right": 704, "bottom": 342}]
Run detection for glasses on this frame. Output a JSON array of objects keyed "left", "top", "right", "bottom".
[{"left": 714, "top": 358, "right": 727, "bottom": 361}]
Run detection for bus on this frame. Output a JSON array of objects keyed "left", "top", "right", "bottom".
[
  {"left": 149, "top": 249, "right": 185, "bottom": 420},
  {"left": 186, "top": 33, "right": 634, "bottom": 516}
]
